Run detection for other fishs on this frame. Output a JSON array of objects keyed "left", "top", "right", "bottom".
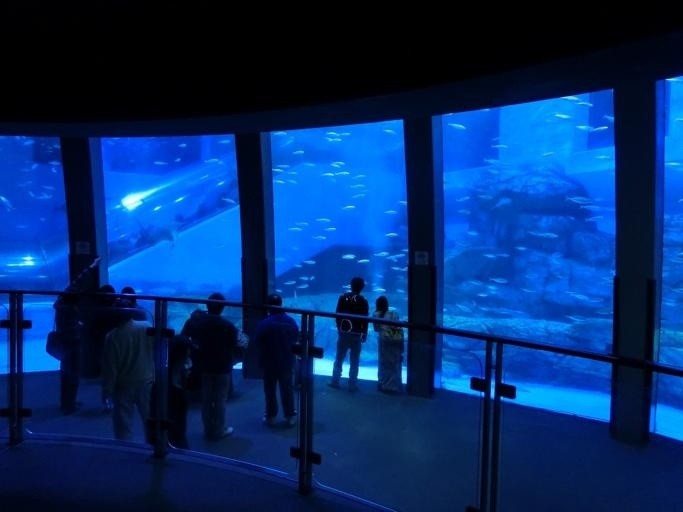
[
  {"left": 442, "top": 90, "right": 616, "bottom": 352},
  {"left": 0, "top": 133, "right": 66, "bottom": 210},
  {"left": 269, "top": 122, "right": 410, "bottom": 322},
  {"left": 149, "top": 137, "right": 237, "bottom": 213}
]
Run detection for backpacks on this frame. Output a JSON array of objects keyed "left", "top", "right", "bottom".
[{"left": 266, "top": 324, "right": 299, "bottom": 368}]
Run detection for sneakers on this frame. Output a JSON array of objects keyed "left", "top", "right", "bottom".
[
  {"left": 330, "top": 379, "right": 360, "bottom": 391},
  {"left": 56, "top": 401, "right": 298, "bottom": 451}
]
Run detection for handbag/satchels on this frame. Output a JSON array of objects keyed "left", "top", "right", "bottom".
[
  {"left": 45, "top": 332, "right": 72, "bottom": 364},
  {"left": 382, "top": 326, "right": 405, "bottom": 345}
]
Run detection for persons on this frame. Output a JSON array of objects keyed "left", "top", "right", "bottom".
[
  {"left": 147, "top": 336, "right": 190, "bottom": 448},
  {"left": 55, "top": 281, "right": 154, "bottom": 442},
  {"left": 182, "top": 292, "right": 239, "bottom": 437},
  {"left": 253, "top": 295, "right": 300, "bottom": 423},
  {"left": 325, "top": 277, "right": 368, "bottom": 391},
  {"left": 372, "top": 296, "right": 405, "bottom": 394}
]
